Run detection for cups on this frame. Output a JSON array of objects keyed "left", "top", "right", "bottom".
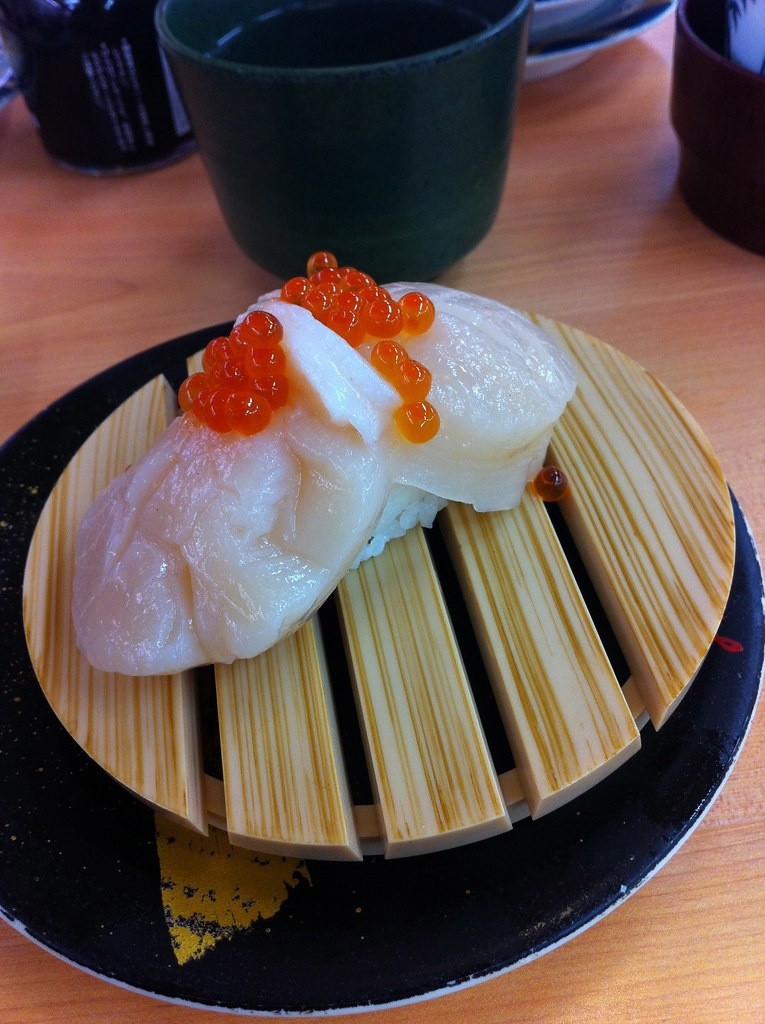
[
  {"left": 156, "top": 0, "right": 533, "bottom": 281},
  {"left": 671, "top": 0, "right": 765, "bottom": 256},
  {"left": 0, "top": 0, "right": 199, "bottom": 176}
]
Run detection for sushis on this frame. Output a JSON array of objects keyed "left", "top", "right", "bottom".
[{"left": 70, "top": 281, "right": 575, "bottom": 676}]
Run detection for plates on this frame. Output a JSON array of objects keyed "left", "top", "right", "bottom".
[
  {"left": 522, "top": 0, "right": 679, "bottom": 81},
  {"left": 0, "top": 321, "right": 765, "bottom": 1016}
]
